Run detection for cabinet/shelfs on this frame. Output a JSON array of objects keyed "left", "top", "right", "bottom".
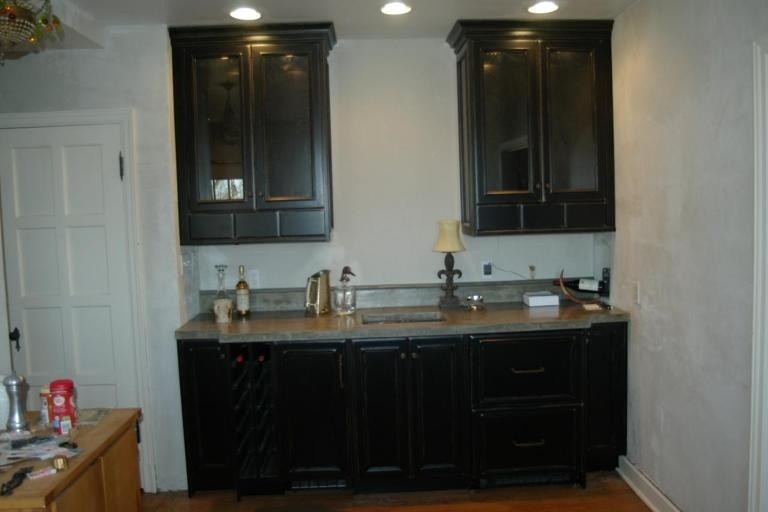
[
  {"left": 0, "top": 409, "right": 142, "bottom": 512},
  {"left": 178, "top": 338, "right": 282, "bottom": 493},
  {"left": 468, "top": 404, "right": 588, "bottom": 488},
  {"left": 582, "top": 324, "right": 625, "bottom": 469},
  {"left": 167, "top": 23, "right": 337, "bottom": 247},
  {"left": 281, "top": 342, "right": 350, "bottom": 492},
  {"left": 351, "top": 336, "right": 465, "bottom": 491},
  {"left": 467, "top": 332, "right": 590, "bottom": 410},
  {"left": 444, "top": 18, "right": 616, "bottom": 236}
]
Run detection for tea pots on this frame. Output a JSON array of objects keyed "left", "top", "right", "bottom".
[{"left": 303, "top": 268, "right": 331, "bottom": 317}]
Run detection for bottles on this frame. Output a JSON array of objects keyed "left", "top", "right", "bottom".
[
  {"left": 213, "top": 264, "right": 228, "bottom": 298},
  {"left": 39, "top": 386, "right": 52, "bottom": 427},
  {"left": 49, "top": 380, "right": 75, "bottom": 434},
  {"left": 235, "top": 264, "right": 250, "bottom": 317},
  {"left": 553, "top": 278, "right": 603, "bottom": 294}
]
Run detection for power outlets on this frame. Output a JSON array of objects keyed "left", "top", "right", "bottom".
[{"left": 482, "top": 262, "right": 493, "bottom": 279}]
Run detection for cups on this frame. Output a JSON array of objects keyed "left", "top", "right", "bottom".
[
  {"left": 213, "top": 299, "right": 233, "bottom": 324},
  {"left": 466, "top": 295, "right": 485, "bottom": 311}
]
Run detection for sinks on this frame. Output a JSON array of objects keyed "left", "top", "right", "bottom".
[{"left": 360, "top": 311, "right": 449, "bottom": 325}]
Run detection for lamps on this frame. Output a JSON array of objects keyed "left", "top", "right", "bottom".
[
  {"left": 432, "top": 220, "right": 465, "bottom": 309},
  {"left": 0, "top": 0, "right": 63, "bottom": 66}
]
